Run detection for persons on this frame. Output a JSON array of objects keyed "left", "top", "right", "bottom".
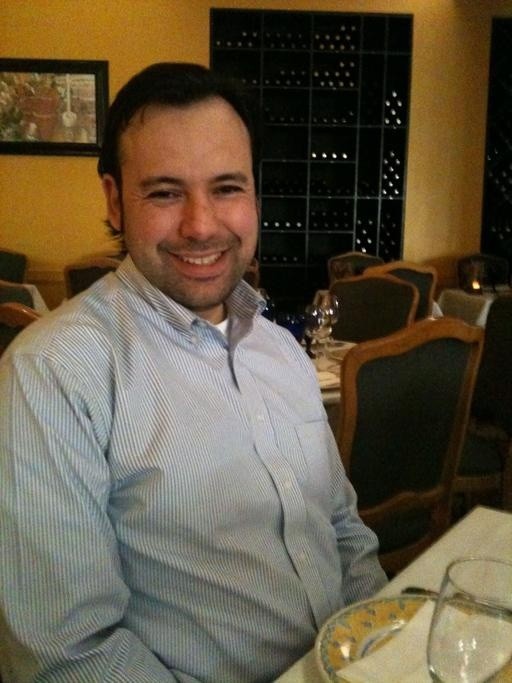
[{"left": 0, "top": 60, "right": 393, "bottom": 681}]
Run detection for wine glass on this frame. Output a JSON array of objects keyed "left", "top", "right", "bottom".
[{"left": 270, "top": 288, "right": 359, "bottom": 391}]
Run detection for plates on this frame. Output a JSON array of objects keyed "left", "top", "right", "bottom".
[{"left": 312, "top": 595, "right": 509, "bottom": 680}]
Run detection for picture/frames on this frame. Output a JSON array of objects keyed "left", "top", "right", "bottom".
[{"left": 0, "top": 52, "right": 113, "bottom": 159}]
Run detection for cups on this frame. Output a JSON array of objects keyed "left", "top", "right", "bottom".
[{"left": 428, "top": 557, "right": 510, "bottom": 680}]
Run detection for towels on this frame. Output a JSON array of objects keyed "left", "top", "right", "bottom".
[{"left": 339, "top": 599, "right": 512, "bottom": 683}]
